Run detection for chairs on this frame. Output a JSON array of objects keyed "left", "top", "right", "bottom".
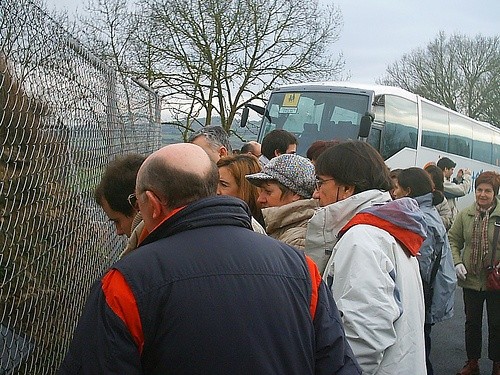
[{"left": 302, "top": 120, "right": 469, "bottom": 159}]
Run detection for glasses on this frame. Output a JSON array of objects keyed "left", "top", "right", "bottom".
[
  {"left": 314, "top": 177, "right": 334, "bottom": 190},
  {"left": 128, "top": 190, "right": 167, "bottom": 212}
]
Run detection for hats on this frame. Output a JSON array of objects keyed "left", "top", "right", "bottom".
[{"left": 244, "top": 154, "right": 317, "bottom": 199}]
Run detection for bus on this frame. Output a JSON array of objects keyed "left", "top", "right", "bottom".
[{"left": 240, "top": 79, "right": 500, "bottom": 220}]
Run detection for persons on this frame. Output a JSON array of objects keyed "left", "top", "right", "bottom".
[{"left": 54, "top": 124, "right": 500, "bottom": 375}]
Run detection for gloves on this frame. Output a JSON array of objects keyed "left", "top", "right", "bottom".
[{"left": 455, "top": 263, "right": 467, "bottom": 281}]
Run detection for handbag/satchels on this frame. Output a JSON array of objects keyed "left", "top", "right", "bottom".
[
  {"left": 422, "top": 280, "right": 434, "bottom": 311},
  {"left": 485, "top": 260, "right": 500, "bottom": 291}
]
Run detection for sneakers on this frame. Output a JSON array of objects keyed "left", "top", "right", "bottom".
[
  {"left": 492, "top": 360, "right": 500, "bottom": 375},
  {"left": 457, "top": 359, "right": 479, "bottom": 375}
]
